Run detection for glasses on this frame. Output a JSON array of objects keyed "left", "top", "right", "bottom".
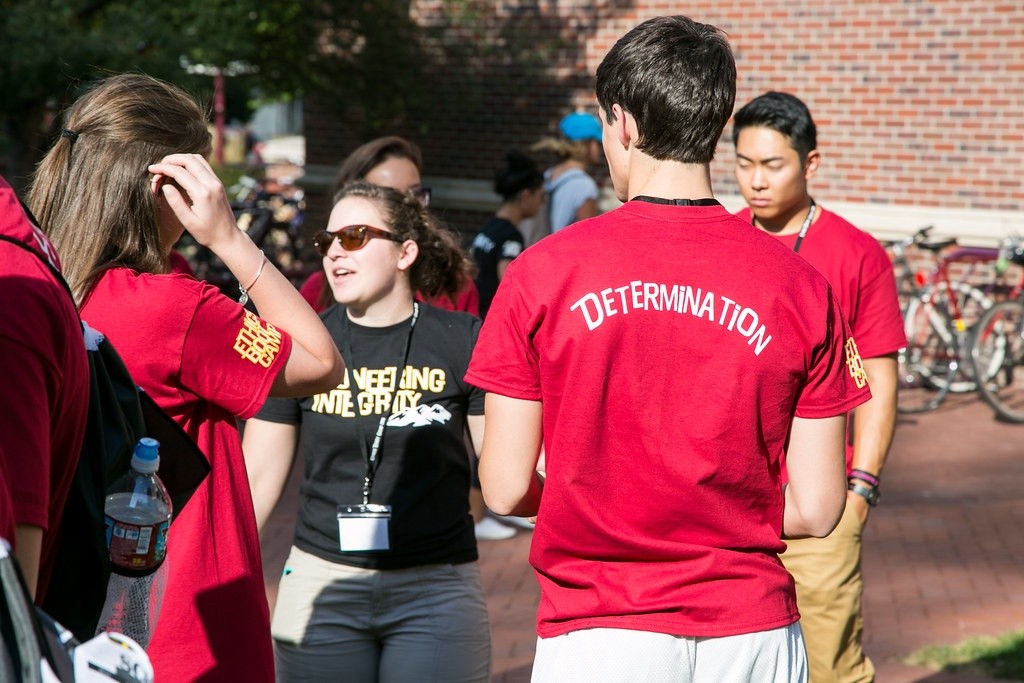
[
  {"left": 407, "top": 185, "right": 433, "bottom": 210},
  {"left": 313, "top": 225, "right": 402, "bottom": 258}
]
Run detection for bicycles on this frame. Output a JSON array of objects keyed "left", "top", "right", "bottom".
[{"left": 879, "top": 223, "right": 1024, "bottom": 427}]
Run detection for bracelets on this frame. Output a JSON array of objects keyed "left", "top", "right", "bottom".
[
  {"left": 229, "top": 248, "right": 266, "bottom": 306},
  {"left": 841, "top": 466, "right": 880, "bottom": 487}
]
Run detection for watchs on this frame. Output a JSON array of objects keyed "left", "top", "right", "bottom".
[{"left": 844, "top": 480, "right": 881, "bottom": 508}]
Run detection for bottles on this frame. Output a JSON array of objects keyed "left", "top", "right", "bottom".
[{"left": 94, "top": 438, "right": 173, "bottom": 650}]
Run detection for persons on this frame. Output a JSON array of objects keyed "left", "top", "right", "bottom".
[
  {"left": 238, "top": 180, "right": 498, "bottom": 682},
  {"left": 469, "top": 112, "right": 606, "bottom": 540},
  {"left": 0, "top": 162, "right": 93, "bottom": 683},
  {"left": 459, "top": 13, "right": 873, "bottom": 683},
  {"left": 725, "top": 89, "right": 904, "bottom": 683},
  {"left": 20, "top": 69, "right": 349, "bottom": 683},
  {"left": 294, "top": 130, "right": 477, "bottom": 321}
]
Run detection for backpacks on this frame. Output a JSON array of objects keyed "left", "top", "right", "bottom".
[
  {"left": 0, "top": 233, "right": 211, "bottom": 642},
  {"left": 516, "top": 171, "right": 588, "bottom": 249}
]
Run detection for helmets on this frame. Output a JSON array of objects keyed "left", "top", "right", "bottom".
[{"left": 560, "top": 114, "right": 604, "bottom": 144}]
